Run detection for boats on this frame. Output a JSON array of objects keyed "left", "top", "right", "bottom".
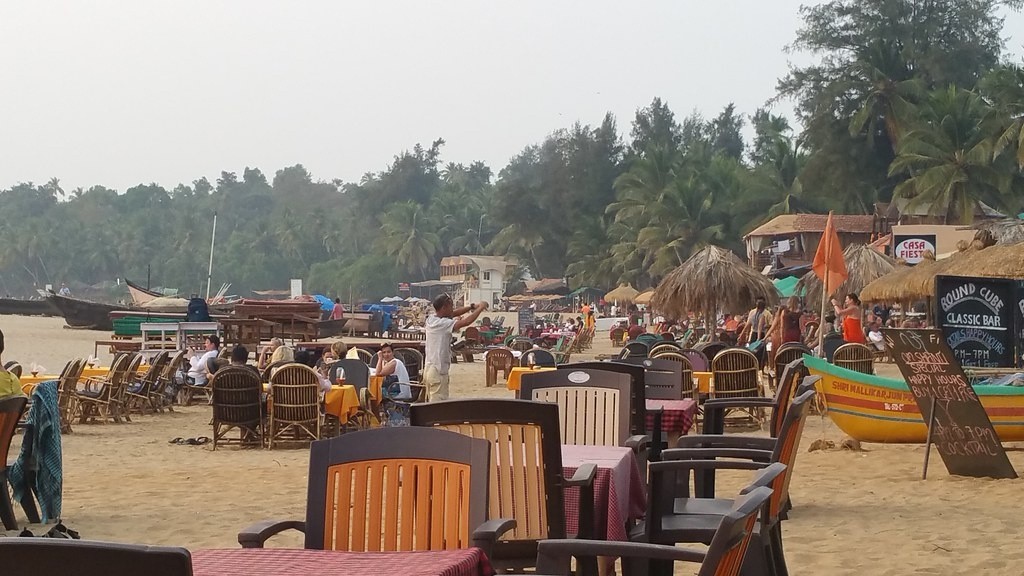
[
  {"left": 78, "top": 365, "right": 150, "bottom": 384},
  {"left": 19, "top": 375, "right": 87, "bottom": 397},
  {"left": 801, "top": 352, "right": 1024, "bottom": 443},
  {"left": 108, "top": 311, "right": 230, "bottom": 336},
  {"left": 43, "top": 294, "right": 244, "bottom": 331}
]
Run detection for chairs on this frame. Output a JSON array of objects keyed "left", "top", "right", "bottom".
[{"left": 0, "top": 296, "right": 930, "bottom": 576}]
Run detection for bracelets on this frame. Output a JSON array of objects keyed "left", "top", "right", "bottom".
[{"left": 471, "top": 303, "right": 475, "bottom": 309}]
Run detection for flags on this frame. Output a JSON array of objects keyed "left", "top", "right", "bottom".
[{"left": 813, "top": 216, "right": 847, "bottom": 298}]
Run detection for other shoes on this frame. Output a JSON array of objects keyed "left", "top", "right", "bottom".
[
  {"left": 42, "top": 523, "right": 80, "bottom": 539},
  {"left": 19, "top": 526, "right": 34, "bottom": 537}
]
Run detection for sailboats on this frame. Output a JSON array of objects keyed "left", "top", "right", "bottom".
[{"left": 124, "top": 211, "right": 245, "bottom": 313}]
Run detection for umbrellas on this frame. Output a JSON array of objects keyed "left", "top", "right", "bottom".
[
  {"left": 509, "top": 242, "right": 1024, "bottom": 343},
  {"left": 381, "top": 296, "right": 420, "bottom": 303}
]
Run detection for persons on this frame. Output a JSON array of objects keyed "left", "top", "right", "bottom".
[
  {"left": 329, "top": 298, "right": 343, "bottom": 338},
  {"left": 483, "top": 296, "right": 927, "bottom": 373},
  {"left": 59, "top": 283, "right": 70, "bottom": 296},
  {"left": 185, "top": 335, "right": 220, "bottom": 386},
  {"left": 0, "top": 330, "right": 22, "bottom": 400},
  {"left": 423, "top": 293, "right": 489, "bottom": 403},
  {"left": 233, "top": 338, "right": 412, "bottom": 423}
]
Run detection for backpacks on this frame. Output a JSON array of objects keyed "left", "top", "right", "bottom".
[{"left": 188, "top": 298, "right": 211, "bottom": 322}]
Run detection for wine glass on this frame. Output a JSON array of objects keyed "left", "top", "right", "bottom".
[
  {"left": 527, "top": 352, "right": 536, "bottom": 370},
  {"left": 336, "top": 367, "right": 346, "bottom": 387},
  {"left": 88, "top": 355, "right": 95, "bottom": 369},
  {"left": 31, "top": 364, "right": 39, "bottom": 378}
]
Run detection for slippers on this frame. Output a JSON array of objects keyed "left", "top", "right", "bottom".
[
  {"left": 196, "top": 437, "right": 212, "bottom": 445},
  {"left": 178, "top": 439, "right": 197, "bottom": 445},
  {"left": 168, "top": 438, "right": 185, "bottom": 443}
]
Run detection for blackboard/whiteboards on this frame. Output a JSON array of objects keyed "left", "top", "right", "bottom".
[
  {"left": 519, "top": 307, "right": 534, "bottom": 336},
  {"left": 878, "top": 327, "right": 1018, "bottom": 480}
]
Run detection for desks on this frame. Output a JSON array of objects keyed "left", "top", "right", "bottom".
[
  {"left": 136, "top": 364, "right": 152, "bottom": 373},
  {"left": 556, "top": 331, "right": 577, "bottom": 344},
  {"left": 19, "top": 375, "right": 60, "bottom": 396},
  {"left": 78, "top": 366, "right": 110, "bottom": 391},
  {"left": 479, "top": 331, "right": 495, "bottom": 347},
  {"left": 334, "top": 375, "right": 384, "bottom": 424},
  {"left": 540, "top": 332, "right": 568, "bottom": 350},
  {"left": 487, "top": 441, "right": 649, "bottom": 576},
  {"left": 644, "top": 398, "right": 701, "bottom": 498},
  {"left": 506, "top": 366, "right": 557, "bottom": 399},
  {"left": 262, "top": 382, "right": 361, "bottom": 439},
  {"left": 691, "top": 371, "right": 716, "bottom": 425},
  {"left": 190, "top": 548, "right": 497, "bottom": 576}
]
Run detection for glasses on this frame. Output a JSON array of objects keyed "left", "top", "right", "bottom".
[{"left": 442, "top": 292, "right": 451, "bottom": 307}]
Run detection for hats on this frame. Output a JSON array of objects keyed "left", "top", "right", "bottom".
[{"left": 204, "top": 335, "right": 220, "bottom": 345}]
